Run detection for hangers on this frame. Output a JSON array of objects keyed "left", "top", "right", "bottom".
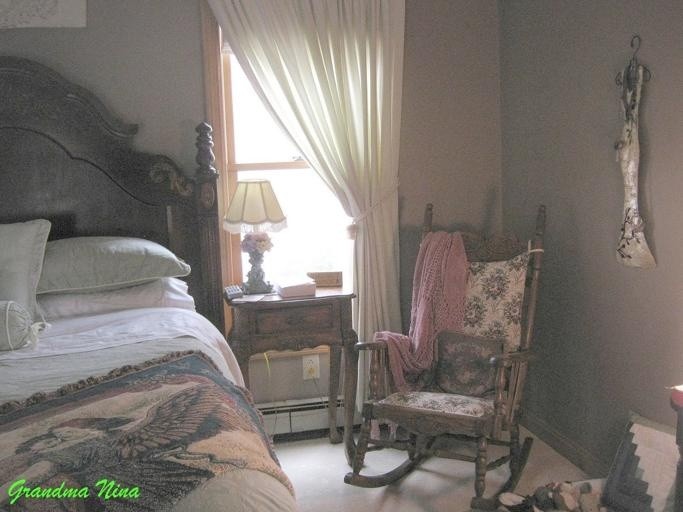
[{"left": 615, "top": 35, "right": 652, "bottom": 85}]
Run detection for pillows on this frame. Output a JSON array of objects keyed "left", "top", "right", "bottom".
[
  {"left": 0, "top": 220, "right": 194, "bottom": 353},
  {"left": 432, "top": 332, "right": 505, "bottom": 398}
]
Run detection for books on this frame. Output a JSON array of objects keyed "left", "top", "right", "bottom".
[{"left": 269, "top": 265, "right": 342, "bottom": 299}]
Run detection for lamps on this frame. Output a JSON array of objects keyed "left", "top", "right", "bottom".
[{"left": 221, "top": 179, "right": 287, "bottom": 295}]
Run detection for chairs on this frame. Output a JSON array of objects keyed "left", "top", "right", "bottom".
[{"left": 344, "top": 203, "right": 551, "bottom": 512}]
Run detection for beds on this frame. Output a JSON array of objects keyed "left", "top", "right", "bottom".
[{"left": 0, "top": 55, "right": 297, "bottom": 512}]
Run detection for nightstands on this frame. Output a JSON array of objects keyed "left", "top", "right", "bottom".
[{"left": 223, "top": 286, "right": 361, "bottom": 469}]
